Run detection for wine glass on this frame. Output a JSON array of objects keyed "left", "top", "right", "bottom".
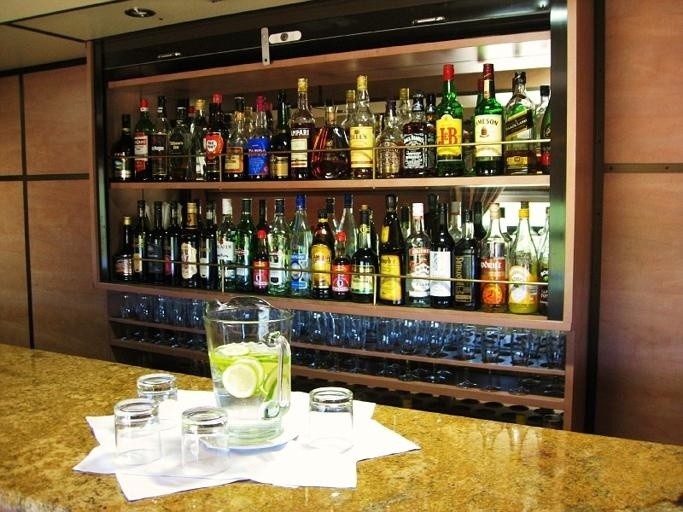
[
  {"left": 294, "top": 309, "right": 567, "bottom": 400},
  {"left": 120, "top": 294, "right": 207, "bottom": 350}
]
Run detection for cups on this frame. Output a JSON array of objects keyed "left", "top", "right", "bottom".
[
  {"left": 203, "top": 295, "right": 293, "bottom": 444},
  {"left": 309, "top": 387, "right": 353, "bottom": 453},
  {"left": 136, "top": 373, "right": 178, "bottom": 424},
  {"left": 115, "top": 398, "right": 163, "bottom": 467},
  {"left": 181, "top": 407, "right": 232, "bottom": 479}
]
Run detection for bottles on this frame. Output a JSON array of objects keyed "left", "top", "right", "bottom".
[
  {"left": 188, "top": 97, "right": 206, "bottom": 181},
  {"left": 223, "top": 112, "right": 236, "bottom": 128},
  {"left": 473, "top": 63, "right": 504, "bottom": 176},
  {"left": 252, "top": 199, "right": 269, "bottom": 295},
  {"left": 312, "top": 104, "right": 351, "bottom": 180},
  {"left": 224, "top": 97, "right": 248, "bottom": 181},
  {"left": 501, "top": 207, "right": 511, "bottom": 246},
  {"left": 535, "top": 84, "right": 550, "bottom": 157},
  {"left": 448, "top": 201, "right": 463, "bottom": 245},
  {"left": 269, "top": 88, "right": 290, "bottom": 180},
  {"left": 110, "top": 112, "right": 132, "bottom": 181},
  {"left": 472, "top": 201, "right": 485, "bottom": 240},
  {"left": 378, "top": 194, "right": 407, "bottom": 306},
  {"left": 186, "top": 108, "right": 194, "bottom": 134},
  {"left": 503, "top": 72, "right": 536, "bottom": 174},
  {"left": 347, "top": 74, "right": 377, "bottom": 179},
  {"left": 429, "top": 203, "right": 454, "bottom": 309},
  {"left": 506, "top": 208, "right": 537, "bottom": 315},
  {"left": 308, "top": 210, "right": 333, "bottom": 300},
  {"left": 398, "top": 90, "right": 429, "bottom": 176},
  {"left": 351, "top": 204, "right": 377, "bottom": 304},
  {"left": 374, "top": 115, "right": 401, "bottom": 176},
  {"left": 406, "top": 201, "right": 433, "bottom": 308},
  {"left": 452, "top": 208, "right": 480, "bottom": 311},
  {"left": 215, "top": 199, "right": 237, "bottom": 292},
  {"left": 168, "top": 107, "right": 193, "bottom": 181},
  {"left": 475, "top": 78, "right": 484, "bottom": 107},
  {"left": 424, "top": 195, "right": 440, "bottom": 242},
  {"left": 397, "top": 88, "right": 410, "bottom": 121},
  {"left": 537, "top": 207, "right": 549, "bottom": 316},
  {"left": 151, "top": 95, "right": 172, "bottom": 182},
  {"left": 511, "top": 200, "right": 538, "bottom": 243},
  {"left": 289, "top": 77, "right": 314, "bottom": 179},
  {"left": 331, "top": 232, "right": 352, "bottom": 301},
  {"left": 237, "top": 197, "right": 255, "bottom": 293},
  {"left": 246, "top": 95, "right": 272, "bottom": 181},
  {"left": 199, "top": 201, "right": 218, "bottom": 290},
  {"left": 133, "top": 199, "right": 150, "bottom": 283},
  {"left": 436, "top": 64, "right": 465, "bottom": 177},
  {"left": 478, "top": 202, "right": 507, "bottom": 311},
  {"left": 148, "top": 201, "right": 164, "bottom": 284},
  {"left": 380, "top": 94, "right": 397, "bottom": 134},
  {"left": 341, "top": 90, "right": 358, "bottom": 128},
  {"left": 290, "top": 192, "right": 313, "bottom": 298},
  {"left": 325, "top": 197, "right": 341, "bottom": 238},
  {"left": 204, "top": 93, "right": 227, "bottom": 182},
  {"left": 267, "top": 198, "right": 292, "bottom": 296},
  {"left": 425, "top": 91, "right": 438, "bottom": 174},
  {"left": 179, "top": 202, "right": 199, "bottom": 289},
  {"left": 164, "top": 201, "right": 179, "bottom": 285},
  {"left": 115, "top": 215, "right": 134, "bottom": 281},
  {"left": 130, "top": 100, "right": 153, "bottom": 181},
  {"left": 540, "top": 98, "right": 550, "bottom": 175},
  {"left": 337, "top": 193, "right": 358, "bottom": 257},
  {"left": 399, "top": 205, "right": 408, "bottom": 238}
]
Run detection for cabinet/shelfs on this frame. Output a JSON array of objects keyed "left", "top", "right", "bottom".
[
  {"left": 108, "top": 314, "right": 568, "bottom": 410},
  {"left": 100, "top": 29, "right": 551, "bottom": 320}
]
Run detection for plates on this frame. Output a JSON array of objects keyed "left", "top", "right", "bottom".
[{"left": 200, "top": 431, "right": 298, "bottom": 449}]
